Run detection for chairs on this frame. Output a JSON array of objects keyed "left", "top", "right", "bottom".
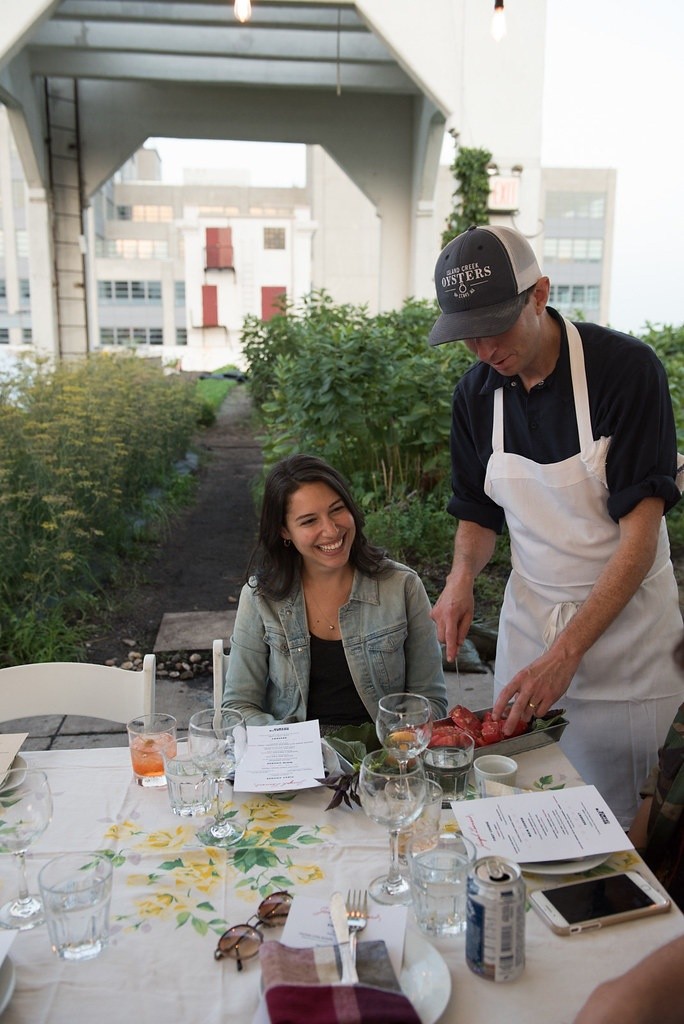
[{"left": 0, "top": 654, "right": 156, "bottom": 750}]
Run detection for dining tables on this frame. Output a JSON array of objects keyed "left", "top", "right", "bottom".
[{"left": 0, "top": 742, "right": 684, "bottom": 1024}]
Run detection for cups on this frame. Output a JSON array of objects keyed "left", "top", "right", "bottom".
[
  {"left": 406, "top": 828, "right": 478, "bottom": 953},
  {"left": 473, "top": 755, "right": 519, "bottom": 799},
  {"left": 125, "top": 712, "right": 178, "bottom": 789},
  {"left": 384, "top": 777, "right": 444, "bottom": 867},
  {"left": 419, "top": 729, "right": 475, "bottom": 809},
  {"left": 38, "top": 851, "right": 115, "bottom": 963},
  {"left": 161, "top": 736, "right": 217, "bottom": 818}
]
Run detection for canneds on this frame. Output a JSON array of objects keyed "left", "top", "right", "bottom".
[{"left": 465, "top": 855, "right": 526, "bottom": 982}]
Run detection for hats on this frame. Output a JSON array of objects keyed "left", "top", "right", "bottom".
[{"left": 428, "top": 224, "right": 543, "bottom": 347}]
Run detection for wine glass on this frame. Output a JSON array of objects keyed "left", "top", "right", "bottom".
[
  {"left": 375, "top": 693, "right": 432, "bottom": 806},
  {"left": 188, "top": 708, "right": 247, "bottom": 847},
  {"left": 357, "top": 749, "right": 428, "bottom": 906},
  {"left": 0, "top": 767, "right": 54, "bottom": 931}
]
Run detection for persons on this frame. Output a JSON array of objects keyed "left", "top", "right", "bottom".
[
  {"left": 429, "top": 223, "right": 684, "bottom": 1024},
  {"left": 221, "top": 455, "right": 448, "bottom": 725}
]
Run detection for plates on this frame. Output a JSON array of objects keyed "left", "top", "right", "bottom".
[
  {"left": 0, "top": 954, "right": 17, "bottom": 1015},
  {"left": 0, "top": 754, "right": 28, "bottom": 792},
  {"left": 519, "top": 853, "right": 614, "bottom": 875}
]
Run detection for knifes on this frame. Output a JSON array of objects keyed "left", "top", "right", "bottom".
[{"left": 330, "top": 891, "right": 359, "bottom": 985}]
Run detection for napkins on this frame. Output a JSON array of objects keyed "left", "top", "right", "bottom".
[{"left": 259, "top": 940, "right": 427, "bottom": 1024}]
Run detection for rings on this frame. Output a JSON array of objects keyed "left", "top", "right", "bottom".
[{"left": 528, "top": 702, "right": 538, "bottom": 710}]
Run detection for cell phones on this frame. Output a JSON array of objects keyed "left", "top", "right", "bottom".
[{"left": 526, "top": 870, "right": 672, "bottom": 937}]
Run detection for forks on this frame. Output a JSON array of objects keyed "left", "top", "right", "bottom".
[{"left": 346, "top": 889, "right": 368, "bottom": 985}]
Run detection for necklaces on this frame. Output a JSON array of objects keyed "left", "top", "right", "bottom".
[{"left": 306, "top": 573, "right": 354, "bottom": 632}]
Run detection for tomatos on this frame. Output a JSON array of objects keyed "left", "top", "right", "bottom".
[{"left": 427, "top": 705, "right": 530, "bottom": 748}]
